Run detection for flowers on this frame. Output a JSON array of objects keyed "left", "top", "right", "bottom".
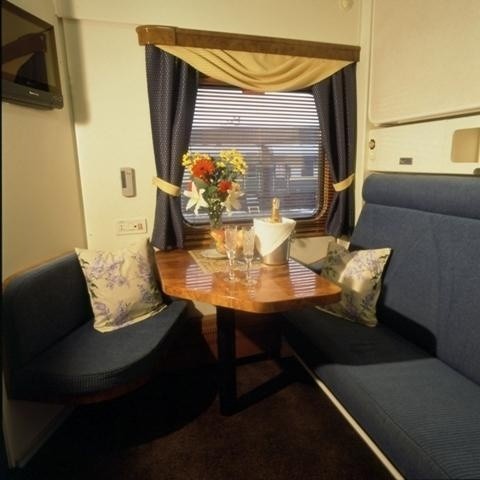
[{"left": 181, "top": 148, "right": 249, "bottom": 228}]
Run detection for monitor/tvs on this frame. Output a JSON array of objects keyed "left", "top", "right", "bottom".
[{"left": 1, "top": 0, "right": 64, "bottom": 109}]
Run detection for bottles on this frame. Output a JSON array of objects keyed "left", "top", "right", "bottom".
[{"left": 270, "top": 198, "right": 281, "bottom": 223}]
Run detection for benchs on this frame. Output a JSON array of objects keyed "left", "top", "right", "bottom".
[
  {"left": 1, "top": 248, "right": 197, "bottom": 407},
  {"left": 282, "top": 172, "right": 479, "bottom": 479}
]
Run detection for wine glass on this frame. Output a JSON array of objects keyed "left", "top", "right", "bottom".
[
  {"left": 223, "top": 223, "right": 241, "bottom": 284},
  {"left": 240, "top": 226, "right": 258, "bottom": 286}
]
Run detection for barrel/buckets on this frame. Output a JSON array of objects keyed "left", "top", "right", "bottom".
[{"left": 252, "top": 216, "right": 297, "bottom": 266}]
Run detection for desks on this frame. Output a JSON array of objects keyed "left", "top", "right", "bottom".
[{"left": 155, "top": 246, "right": 343, "bottom": 417}]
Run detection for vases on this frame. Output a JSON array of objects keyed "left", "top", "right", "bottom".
[{"left": 208, "top": 215, "right": 222, "bottom": 247}]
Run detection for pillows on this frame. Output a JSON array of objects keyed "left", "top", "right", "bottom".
[
  {"left": 316, "top": 237, "right": 393, "bottom": 329},
  {"left": 74, "top": 234, "right": 169, "bottom": 334}
]
